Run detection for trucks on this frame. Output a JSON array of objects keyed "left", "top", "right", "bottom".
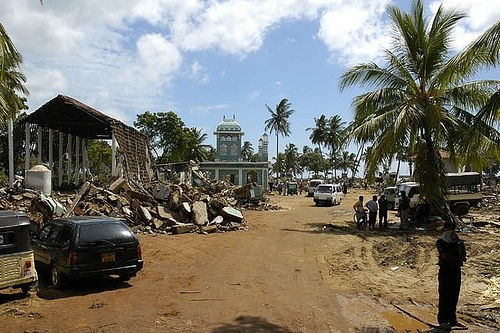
[{"left": 378, "top": 171, "right": 483, "bottom": 217}]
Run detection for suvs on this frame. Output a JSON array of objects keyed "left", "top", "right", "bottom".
[{"left": 30, "top": 215, "right": 144, "bottom": 289}]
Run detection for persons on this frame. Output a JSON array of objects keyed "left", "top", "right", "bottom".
[
  {"left": 436, "top": 220, "right": 467, "bottom": 330},
  {"left": 267, "top": 177, "right": 406, "bottom": 196},
  {"left": 353, "top": 195, "right": 390, "bottom": 230},
  {"left": 398, "top": 191, "right": 410, "bottom": 230}
]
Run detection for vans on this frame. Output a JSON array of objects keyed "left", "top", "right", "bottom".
[
  {"left": 313, "top": 184, "right": 343, "bottom": 206},
  {"left": 307, "top": 179, "right": 325, "bottom": 196}
]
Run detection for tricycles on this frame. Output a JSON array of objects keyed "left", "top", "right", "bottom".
[
  {"left": 0, "top": 210, "right": 38, "bottom": 297},
  {"left": 287, "top": 182, "right": 298, "bottom": 197}
]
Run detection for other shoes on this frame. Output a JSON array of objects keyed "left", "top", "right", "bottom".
[
  {"left": 449, "top": 321, "right": 468, "bottom": 330},
  {"left": 439, "top": 322, "right": 451, "bottom": 332}
]
